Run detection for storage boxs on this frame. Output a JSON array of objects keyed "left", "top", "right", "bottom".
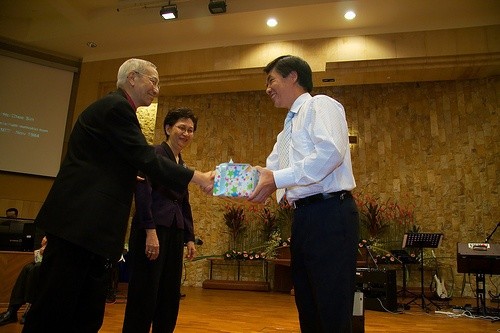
[{"left": 213, "top": 159, "right": 259, "bottom": 199}]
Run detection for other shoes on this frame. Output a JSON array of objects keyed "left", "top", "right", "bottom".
[{"left": 0, "top": 311, "right": 18, "bottom": 326}]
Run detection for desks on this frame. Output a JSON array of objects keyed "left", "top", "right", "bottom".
[{"left": 202, "top": 257, "right": 271, "bottom": 291}]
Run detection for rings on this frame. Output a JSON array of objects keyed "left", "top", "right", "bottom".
[
  {"left": 148, "top": 251, "right": 151, "bottom": 254},
  {"left": 152, "top": 252, "right": 156, "bottom": 254}
]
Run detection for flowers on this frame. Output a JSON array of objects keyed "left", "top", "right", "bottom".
[
  {"left": 223, "top": 202, "right": 291, "bottom": 260},
  {"left": 359, "top": 199, "right": 421, "bottom": 263}
]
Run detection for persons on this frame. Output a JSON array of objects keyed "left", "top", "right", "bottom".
[
  {"left": 0, "top": 236, "right": 47, "bottom": 326},
  {"left": 6, "top": 208, "right": 18, "bottom": 219},
  {"left": 199, "top": 55, "right": 361, "bottom": 333},
  {"left": 22, "top": 58, "right": 215, "bottom": 332},
  {"left": 121, "top": 107, "right": 198, "bottom": 333}
]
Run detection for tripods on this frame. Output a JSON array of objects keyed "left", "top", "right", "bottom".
[{"left": 402, "top": 232, "right": 444, "bottom": 312}]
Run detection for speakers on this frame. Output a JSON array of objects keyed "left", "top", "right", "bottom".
[
  {"left": 456, "top": 241, "right": 500, "bottom": 275},
  {"left": 354, "top": 269, "right": 398, "bottom": 312}
]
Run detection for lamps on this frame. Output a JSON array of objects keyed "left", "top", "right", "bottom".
[
  {"left": 208, "top": 0, "right": 227, "bottom": 14},
  {"left": 160, "top": 0, "right": 178, "bottom": 20}
]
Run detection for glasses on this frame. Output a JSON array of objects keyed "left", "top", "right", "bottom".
[
  {"left": 133, "top": 70, "right": 162, "bottom": 90},
  {"left": 173, "top": 123, "right": 196, "bottom": 134}
]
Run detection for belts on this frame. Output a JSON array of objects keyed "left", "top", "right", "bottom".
[{"left": 292, "top": 190, "right": 351, "bottom": 209}]
